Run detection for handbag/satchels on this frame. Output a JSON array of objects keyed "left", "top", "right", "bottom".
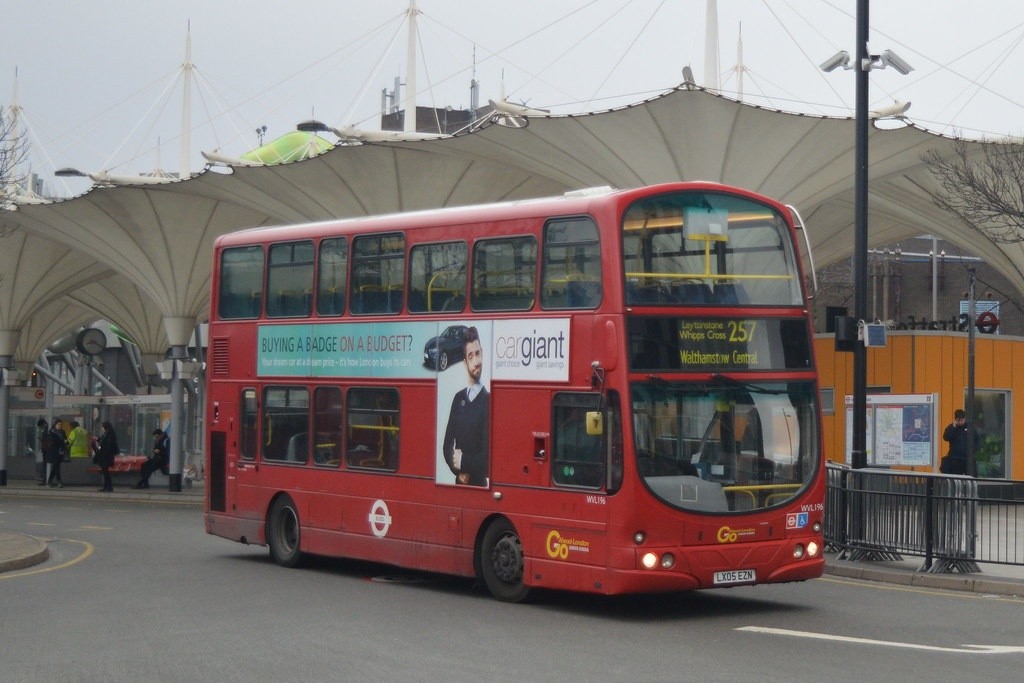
[
  {"left": 941, "top": 455, "right": 951, "bottom": 473},
  {"left": 93, "top": 440, "right": 103, "bottom": 455},
  {"left": 63, "top": 448, "right": 72, "bottom": 462}
]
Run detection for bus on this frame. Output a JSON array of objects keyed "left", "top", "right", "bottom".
[{"left": 203, "top": 183, "right": 827, "bottom": 603}]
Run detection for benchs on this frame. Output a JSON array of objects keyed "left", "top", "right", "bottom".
[
  {"left": 263, "top": 411, "right": 401, "bottom": 469},
  {"left": 87, "top": 455, "right": 147, "bottom": 487}
]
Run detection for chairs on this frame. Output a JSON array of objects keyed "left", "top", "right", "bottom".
[{"left": 252, "top": 265, "right": 752, "bottom": 306}]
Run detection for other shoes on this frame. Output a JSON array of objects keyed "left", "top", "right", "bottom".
[
  {"left": 98, "top": 487, "right": 113, "bottom": 492},
  {"left": 136, "top": 482, "right": 149, "bottom": 489},
  {"left": 48, "top": 482, "right": 55, "bottom": 487},
  {"left": 58, "top": 481, "right": 65, "bottom": 488}
]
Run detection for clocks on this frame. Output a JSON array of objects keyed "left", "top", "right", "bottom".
[{"left": 77, "top": 328, "right": 107, "bottom": 355}]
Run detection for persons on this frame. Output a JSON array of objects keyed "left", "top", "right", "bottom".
[
  {"left": 443, "top": 326, "right": 490, "bottom": 487},
  {"left": 37, "top": 419, "right": 170, "bottom": 492},
  {"left": 943, "top": 409, "right": 979, "bottom": 478}
]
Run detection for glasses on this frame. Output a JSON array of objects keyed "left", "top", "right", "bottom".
[{"left": 955, "top": 418, "right": 964, "bottom": 421}]
